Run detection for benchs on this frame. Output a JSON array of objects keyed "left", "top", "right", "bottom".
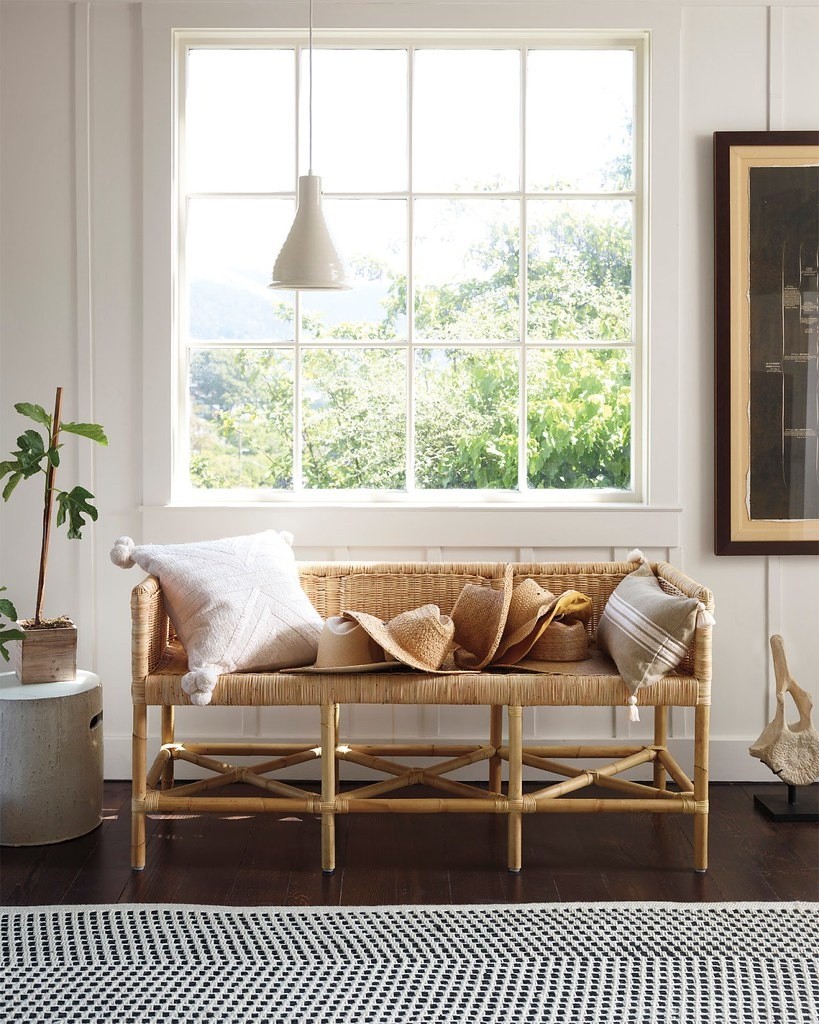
[{"left": 131, "top": 561, "right": 714, "bottom": 872}]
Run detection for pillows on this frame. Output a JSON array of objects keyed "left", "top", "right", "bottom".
[
  {"left": 111, "top": 529, "right": 325, "bottom": 706},
  {"left": 590, "top": 548, "right": 716, "bottom": 722}
]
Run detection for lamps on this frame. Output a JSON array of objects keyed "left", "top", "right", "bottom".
[{"left": 274, "top": 1, "right": 344, "bottom": 290}]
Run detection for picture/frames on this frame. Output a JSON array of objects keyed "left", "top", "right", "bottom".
[{"left": 714, "top": 131, "right": 819, "bottom": 555}]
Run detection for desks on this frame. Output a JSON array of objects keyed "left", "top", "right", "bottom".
[{"left": 0, "top": 670, "right": 103, "bottom": 846}]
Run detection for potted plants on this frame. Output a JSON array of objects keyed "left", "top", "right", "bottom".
[{"left": 0, "top": 388, "right": 108, "bottom": 684}]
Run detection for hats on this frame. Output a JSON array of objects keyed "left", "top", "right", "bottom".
[
  {"left": 488, "top": 578, "right": 617, "bottom": 676},
  {"left": 450, "top": 563, "right": 513, "bottom": 670},
  {"left": 279, "top": 617, "right": 401, "bottom": 674},
  {"left": 343, "top": 604, "right": 482, "bottom": 674}
]
